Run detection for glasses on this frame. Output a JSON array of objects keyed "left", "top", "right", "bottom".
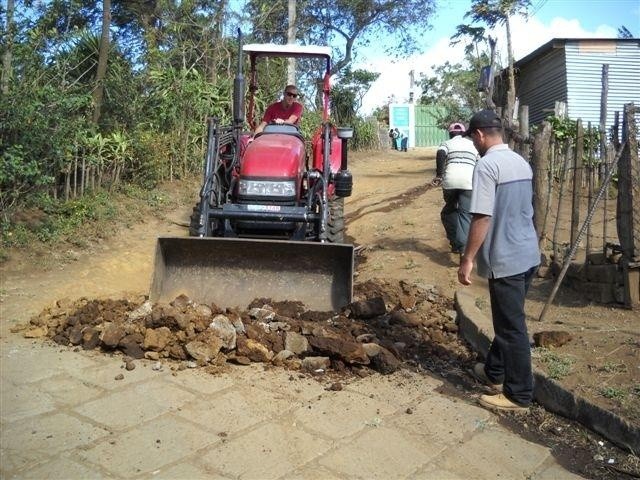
[{"left": 285, "top": 92, "right": 297, "bottom": 98}]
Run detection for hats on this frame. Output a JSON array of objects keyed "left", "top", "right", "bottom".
[
  {"left": 448, "top": 123, "right": 465, "bottom": 133},
  {"left": 465, "top": 109, "right": 502, "bottom": 137}
]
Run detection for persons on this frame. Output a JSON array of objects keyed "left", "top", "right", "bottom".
[
  {"left": 244, "top": 85, "right": 304, "bottom": 145},
  {"left": 431, "top": 122, "right": 483, "bottom": 268},
  {"left": 388, "top": 128, "right": 399, "bottom": 150},
  {"left": 457, "top": 108, "right": 543, "bottom": 412},
  {"left": 394, "top": 127, "right": 409, "bottom": 152}
]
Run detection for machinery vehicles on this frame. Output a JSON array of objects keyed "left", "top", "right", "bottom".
[{"left": 145, "top": 26, "right": 356, "bottom": 317}]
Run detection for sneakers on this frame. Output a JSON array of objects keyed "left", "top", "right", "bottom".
[
  {"left": 473, "top": 363, "right": 505, "bottom": 391},
  {"left": 478, "top": 392, "right": 530, "bottom": 413}
]
